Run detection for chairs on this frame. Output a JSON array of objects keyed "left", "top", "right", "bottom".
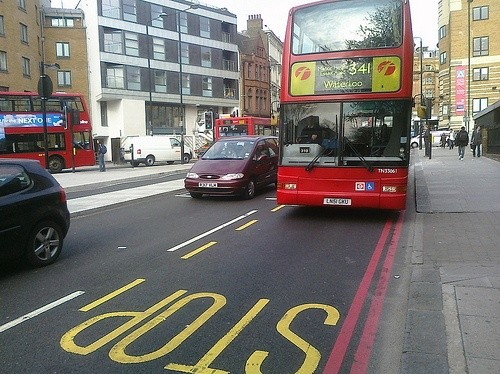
[
  {"left": 244, "top": 142, "right": 254, "bottom": 153},
  {"left": 235, "top": 145, "right": 246, "bottom": 157},
  {"left": 227, "top": 142, "right": 239, "bottom": 152}
]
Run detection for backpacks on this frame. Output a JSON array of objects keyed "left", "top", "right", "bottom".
[{"left": 98, "top": 144, "right": 107, "bottom": 154}]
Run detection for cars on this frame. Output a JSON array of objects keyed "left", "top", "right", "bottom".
[{"left": 0, "top": 158, "right": 71, "bottom": 267}]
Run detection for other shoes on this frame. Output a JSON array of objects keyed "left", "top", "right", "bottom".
[
  {"left": 473, "top": 155, "right": 475, "bottom": 157},
  {"left": 459, "top": 155, "right": 464, "bottom": 160},
  {"left": 99, "top": 170, "right": 105, "bottom": 172},
  {"left": 478, "top": 154, "right": 480, "bottom": 157}
]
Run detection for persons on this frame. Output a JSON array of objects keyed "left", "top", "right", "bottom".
[
  {"left": 96, "top": 139, "right": 105, "bottom": 172},
  {"left": 456, "top": 127, "right": 469, "bottom": 160},
  {"left": 305, "top": 130, "right": 322, "bottom": 145},
  {"left": 72, "top": 134, "right": 83, "bottom": 149},
  {"left": 440, "top": 133, "right": 446, "bottom": 148},
  {"left": 423, "top": 124, "right": 430, "bottom": 157},
  {"left": 454, "top": 131, "right": 459, "bottom": 140},
  {"left": 471, "top": 125, "right": 482, "bottom": 157},
  {"left": 449, "top": 129, "right": 455, "bottom": 150}
]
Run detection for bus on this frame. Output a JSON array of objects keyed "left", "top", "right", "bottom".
[
  {"left": 214, "top": 115, "right": 279, "bottom": 140},
  {"left": 0, "top": 89, "right": 97, "bottom": 174},
  {"left": 271, "top": 0, "right": 428, "bottom": 211}
]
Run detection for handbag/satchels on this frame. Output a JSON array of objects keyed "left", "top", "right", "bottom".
[{"left": 470, "top": 143, "right": 473, "bottom": 149}]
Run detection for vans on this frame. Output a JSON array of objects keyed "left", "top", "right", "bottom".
[
  {"left": 121, "top": 135, "right": 194, "bottom": 167},
  {"left": 184, "top": 134, "right": 281, "bottom": 200},
  {"left": 410, "top": 131, "right": 451, "bottom": 148}
]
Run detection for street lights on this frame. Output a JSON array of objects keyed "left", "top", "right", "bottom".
[
  {"left": 177, "top": 3, "right": 200, "bottom": 166},
  {"left": 146, "top": 13, "right": 169, "bottom": 135}
]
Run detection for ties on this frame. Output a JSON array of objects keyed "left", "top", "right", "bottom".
[{"left": 309, "top": 126, "right": 311, "bottom": 129}]
